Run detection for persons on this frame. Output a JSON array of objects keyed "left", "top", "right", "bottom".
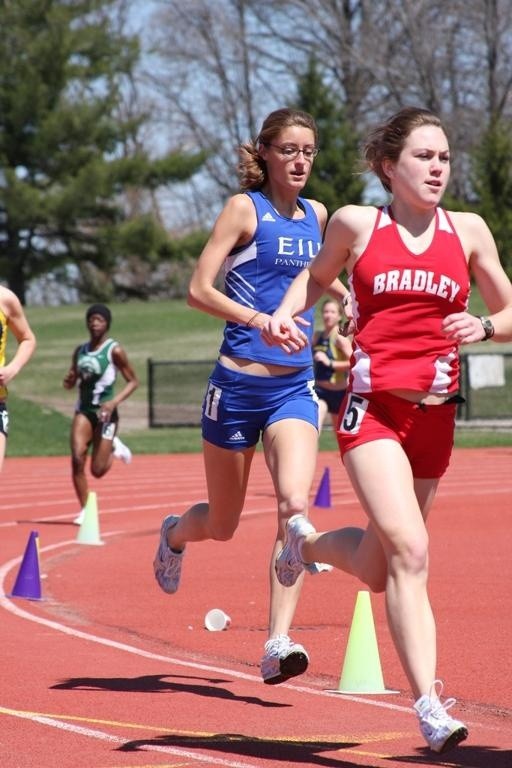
[
  {"left": 257, "top": 104, "right": 511, "bottom": 754},
  {"left": 62, "top": 304, "right": 141, "bottom": 527},
  {"left": 311, "top": 299, "right": 356, "bottom": 440},
  {"left": 152, "top": 110, "right": 357, "bottom": 685},
  {"left": 0, "top": 279, "right": 39, "bottom": 476}
]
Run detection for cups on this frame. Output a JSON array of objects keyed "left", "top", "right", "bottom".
[{"left": 204, "top": 607, "right": 232, "bottom": 632}]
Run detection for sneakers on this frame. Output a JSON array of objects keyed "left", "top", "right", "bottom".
[
  {"left": 413, "top": 694, "right": 468, "bottom": 753},
  {"left": 154, "top": 516, "right": 186, "bottom": 593},
  {"left": 73, "top": 508, "right": 83, "bottom": 526},
  {"left": 261, "top": 636, "right": 308, "bottom": 684},
  {"left": 113, "top": 436, "right": 131, "bottom": 464},
  {"left": 275, "top": 515, "right": 316, "bottom": 586}
]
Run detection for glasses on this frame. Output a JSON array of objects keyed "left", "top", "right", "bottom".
[{"left": 266, "top": 142, "right": 318, "bottom": 158}]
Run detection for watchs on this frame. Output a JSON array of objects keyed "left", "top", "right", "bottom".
[{"left": 475, "top": 316, "right": 497, "bottom": 343}]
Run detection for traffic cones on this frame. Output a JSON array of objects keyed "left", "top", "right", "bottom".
[
  {"left": 69, "top": 490, "right": 106, "bottom": 547},
  {"left": 311, "top": 467, "right": 332, "bottom": 511},
  {"left": 323, "top": 590, "right": 399, "bottom": 697},
  {"left": 5, "top": 529, "right": 47, "bottom": 604}
]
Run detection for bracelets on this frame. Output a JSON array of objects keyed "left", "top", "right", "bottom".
[
  {"left": 246, "top": 312, "right": 263, "bottom": 328},
  {"left": 342, "top": 292, "right": 351, "bottom": 305},
  {"left": 329, "top": 359, "right": 332, "bottom": 368}
]
